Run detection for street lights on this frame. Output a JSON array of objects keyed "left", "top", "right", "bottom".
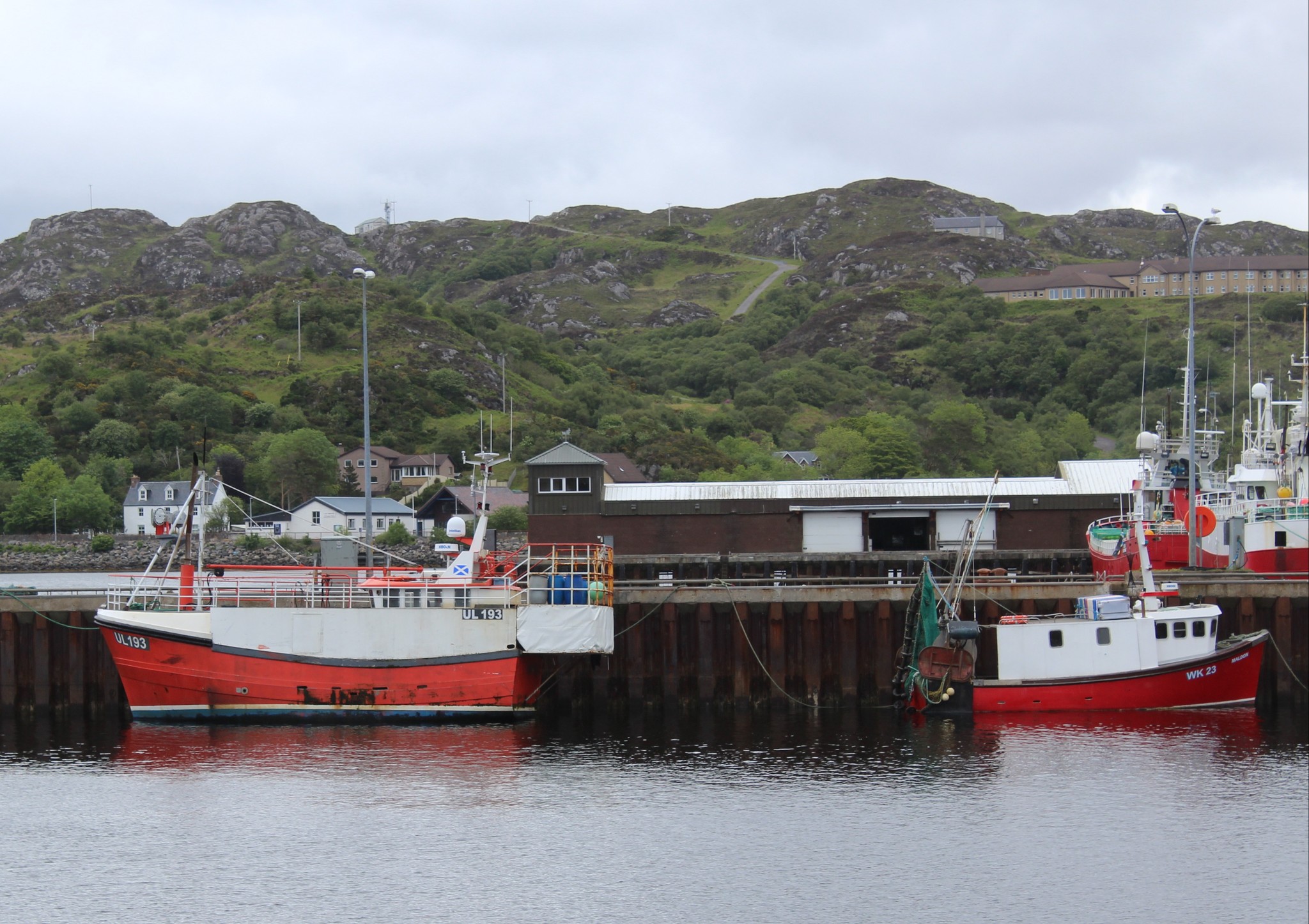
[
  {"left": 353, "top": 266, "right": 376, "bottom": 577},
  {"left": 1163, "top": 204, "right": 1220, "bottom": 567}
]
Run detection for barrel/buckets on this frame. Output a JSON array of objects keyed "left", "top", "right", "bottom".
[
  {"left": 565, "top": 574, "right": 584, "bottom": 604},
  {"left": 548, "top": 574, "right": 565, "bottom": 604},
  {"left": 529, "top": 574, "right": 548, "bottom": 605},
  {"left": 582, "top": 578, "right": 587, "bottom": 605}
]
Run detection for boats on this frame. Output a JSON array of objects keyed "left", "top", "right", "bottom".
[
  {"left": 94, "top": 540, "right": 617, "bottom": 722},
  {"left": 969, "top": 484, "right": 1271, "bottom": 715},
  {"left": 1085, "top": 263, "right": 1308, "bottom": 583}
]
